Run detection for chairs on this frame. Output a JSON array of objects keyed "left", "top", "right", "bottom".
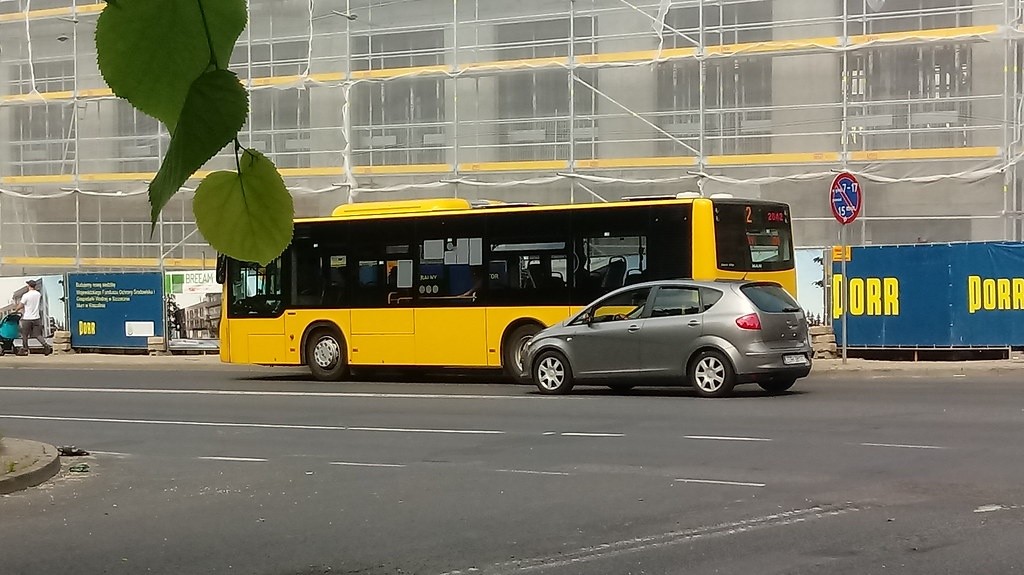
[
  {"left": 522, "top": 259, "right": 567, "bottom": 300},
  {"left": 320, "top": 275, "right": 329, "bottom": 306},
  {"left": 604, "top": 255, "right": 644, "bottom": 291}
]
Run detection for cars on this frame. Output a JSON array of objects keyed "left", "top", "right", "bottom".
[{"left": 520, "top": 280, "right": 814, "bottom": 398}]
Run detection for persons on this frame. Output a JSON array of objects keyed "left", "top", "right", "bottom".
[
  {"left": 619, "top": 298, "right": 645, "bottom": 320},
  {"left": 14, "top": 280, "right": 53, "bottom": 356},
  {"left": 457, "top": 269, "right": 484, "bottom": 307}
]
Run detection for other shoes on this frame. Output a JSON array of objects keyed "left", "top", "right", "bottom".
[
  {"left": 16, "top": 350, "right": 28, "bottom": 356},
  {"left": 44, "top": 344, "right": 50, "bottom": 356}
]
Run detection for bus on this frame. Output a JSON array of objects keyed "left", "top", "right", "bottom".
[{"left": 214, "top": 198, "right": 798, "bottom": 386}]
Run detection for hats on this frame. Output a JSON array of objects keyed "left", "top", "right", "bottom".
[{"left": 26, "top": 280, "right": 36, "bottom": 288}]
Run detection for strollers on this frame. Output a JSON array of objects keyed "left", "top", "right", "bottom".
[{"left": 0, "top": 312, "right": 23, "bottom": 357}]
